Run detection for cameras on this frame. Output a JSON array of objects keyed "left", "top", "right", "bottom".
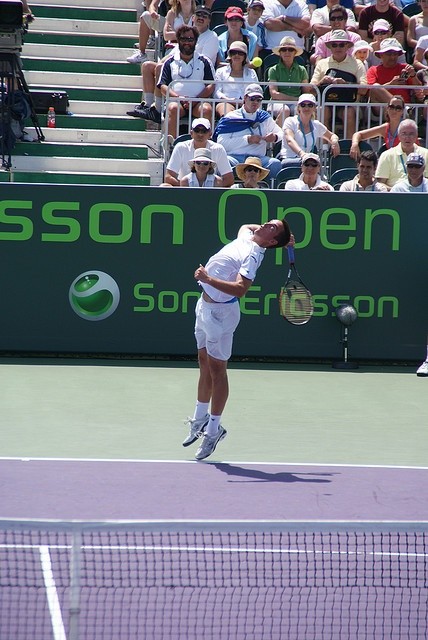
[{"left": 0, "top": 0, "right": 35, "bottom": 53}]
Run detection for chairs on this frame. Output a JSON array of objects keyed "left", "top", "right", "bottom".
[
  {"left": 330, "top": 154, "right": 360, "bottom": 178},
  {"left": 265, "top": 86, "right": 272, "bottom": 100},
  {"left": 170, "top": 135, "right": 214, "bottom": 154},
  {"left": 263, "top": 65, "right": 270, "bottom": 85},
  {"left": 273, "top": 141, "right": 317, "bottom": 155},
  {"left": 277, "top": 167, "right": 328, "bottom": 184},
  {"left": 401, "top": 3, "right": 423, "bottom": 18},
  {"left": 277, "top": 182, "right": 285, "bottom": 189},
  {"left": 378, "top": 139, "right": 425, "bottom": 157},
  {"left": 211, "top": 24, "right": 228, "bottom": 37},
  {"left": 334, "top": 184, "right": 342, "bottom": 190},
  {"left": 234, "top": 180, "right": 270, "bottom": 190},
  {"left": 329, "top": 168, "right": 360, "bottom": 186},
  {"left": 327, "top": 139, "right": 374, "bottom": 162},
  {"left": 261, "top": 53, "right": 307, "bottom": 79}
]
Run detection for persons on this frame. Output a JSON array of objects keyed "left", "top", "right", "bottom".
[
  {"left": 350, "top": 95, "right": 419, "bottom": 161},
  {"left": 352, "top": 40, "right": 373, "bottom": 70},
  {"left": 163, "top": 1, "right": 197, "bottom": 57},
  {"left": 315, "top": 4, "right": 362, "bottom": 61},
  {"left": 309, "top": 28, "right": 368, "bottom": 140},
  {"left": 182, "top": 219, "right": 295, "bottom": 460},
  {"left": 179, "top": 148, "right": 222, "bottom": 187},
  {"left": 126, "top": 1, "right": 172, "bottom": 64},
  {"left": 413, "top": 35, "right": 428, "bottom": 70},
  {"left": 0, "top": 1, "right": 31, "bottom": 140},
  {"left": 390, "top": 152, "right": 427, "bottom": 195},
  {"left": 367, "top": 37, "right": 422, "bottom": 116},
  {"left": 285, "top": 153, "right": 334, "bottom": 192},
  {"left": 126, "top": 5, "right": 220, "bottom": 123},
  {"left": 375, "top": 118, "right": 428, "bottom": 187},
  {"left": 156, "top": 26, "right": 215, "bottom": 139},
  {"left": 129, "top": 0, "right": 157, "bottom": 49},
  {"left": 212, "top": 84, "right": 282, "bottom": 179},
  {"left": 229, "top": 155, "right": 269, "bottom": 190},
  {"left": 352, "top": 0, "right": 396, "bottom": 20},
  {"left": 261, "top": 1, "right": 310, "bottom": 50},
  {"left": 218, "top": 6, "right": 259, "bottom": 69},
  {"left": 311, "top": 0, "right": 359, "bottom": 47},
  {"left": 266, "top": 36, "right": 310, "bottom": 128},
  {"left": 359, "top": 0, "right": 405, "bottom": 46},
  {"left": 407, "top": 0, "right": 428, "bottom": 47},
  {"left": 339, "top": 150, "right": 388, "bottom": 193},
  {"left": 215, "top": 41, "right": 260, "bottom": 116},
  {"left": 283, "top": 93, "right": 342, "bottom": 160},
  {"left": 204, "top": 0, "right": 215, "bottom": 9},
  {"left": 242, "top": 0, "right": 268, "bottom": 59},
  {"left": 160, "top": 117, "right": 235, "bottom": 187},
  {"left": 368, "top": 18, "right": 406, "bottom": 66}
]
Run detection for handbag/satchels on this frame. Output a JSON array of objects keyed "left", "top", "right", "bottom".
[{"left": 316, "top": 68, "right": 357, "bottom": 102}]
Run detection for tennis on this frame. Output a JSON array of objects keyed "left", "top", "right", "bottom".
[{"left": 253, "top": 56, "right": 263, "bottom": 67}]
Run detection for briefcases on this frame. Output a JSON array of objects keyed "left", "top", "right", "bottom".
[{"left": 25, "top": 89, "right": 69, "bottom": 115}]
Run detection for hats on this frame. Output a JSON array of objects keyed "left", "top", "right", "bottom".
[
  {"left": 236, "top": 156, "right": 270, "bottom": 181},
  {"left": 191, "top": 118, "right": 211, "bottom": 130},
  {"left": 297, "top": 93, "right": 316, "bottom": 104},
  {"left": 194, "top": 5, "right": 211, "bottom": 15},
  {"left": 352, "top": 40, "right": 373, "bottom": 59},
  {"left": 373, "top": 18, "right": 390, "bottom": 32},
  {"left": 324, "top": 29, "right": 354, "bottom": 50},
  {"left": 227, "top": 41, "right": 248, "bottom": 58},
  {"left": 406, "top": 153, "right": 425, "bottom": 166},
  {"left": 272, "top": 36, "right": 303, "bottom": 57},
  {"left": 373, "top": 38, "right": 407, "bottom": 59},
  {"left": 224, "top": 6, "right": 244, "bottom": 19},
  {"left": 187, "top": 148, "right": 217, "bottom": 169},
  {"left": 244, "top": 84, "right": 263, "bottom": 98},
  {"left": 248, "top": 1, "right": 265, "bottom": 10},
  {"left": 302, "top": 152, "right": 321, "bottom": 164}
]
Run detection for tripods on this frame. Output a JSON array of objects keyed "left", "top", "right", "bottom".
[{"left": 1, "top": 54, "right": 46, "bottom": 167}]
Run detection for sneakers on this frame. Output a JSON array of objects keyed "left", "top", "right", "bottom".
[
  {"left": 182, "top": 412, "right": 210, "bottom": 447},
  {"left": 135, "top": 38, "right": 155, "bottom": 49},
  {"left": 127, "top": 53, "right": 149, "bottom": 63},
  {"left": 195, "top": 424, "right": 227, "bottom": 460},
  {"left": 126, "top": 101, "right": 166, "bottom": 124},
  {"left": 403, "top": 106, "right": 416, "bottom": 120},
  {"left": 416, "top": 356, "right": 428, "bottom": 376}
]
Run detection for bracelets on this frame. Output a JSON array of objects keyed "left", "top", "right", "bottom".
[
  {"left": 409, "top": 73, "right": 416, "bottom": 79},
  {"left": 296, "top": 149, "right": 302, "bottom": 154}
]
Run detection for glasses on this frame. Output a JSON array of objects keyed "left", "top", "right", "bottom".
[
  {"left": 331, "top": 43, "right": 349, "bottom": 48},
  {"left": 247, "top": 167, "right": 258, "bottom": 173},
  {"left": 407, "top": 164, "right": 423, "bottom": 169},
  {"left": 300, "top": 103, "right": 313, "bottom": 108},
  {"left": 374, "top": 31, "right": 387, "bottom": 35},
  {"left": 303, "top": 163, "right": 318, "bottom": 167},
  {"left": 196, "top": 13, "right": 209, "bottom": 18},
  {"left": 249, "top": 96, "right": 262, "bottom": 102},
  {"left": 231, "top": 51, "right": 244, "bottom": 55},
  {"left": 388, "top": 105, "right": 403, "bottom": 110},
  {"left": 227, "top": 18, "right": 240, "bottom": 22},
  {"left": 196, "top": 161, "right": 209, "bottom": 166},
  {"left": 330, "top": 16, "right": 346, "bottom": 21},
  {"left": 193, "top": 128, "right": 209, "bottom": 134},
  {"left": 385, "top": 51, "right": 399, "bottom": 56},
  {"left": 280, "top": 48, "right": 295, "bottom": 52},
  {"left": 400, "top": 134, "right": 415, "bottom": 138},
  {"left": 179, "top": 37, "right": 194, "bottom": 42}
]
